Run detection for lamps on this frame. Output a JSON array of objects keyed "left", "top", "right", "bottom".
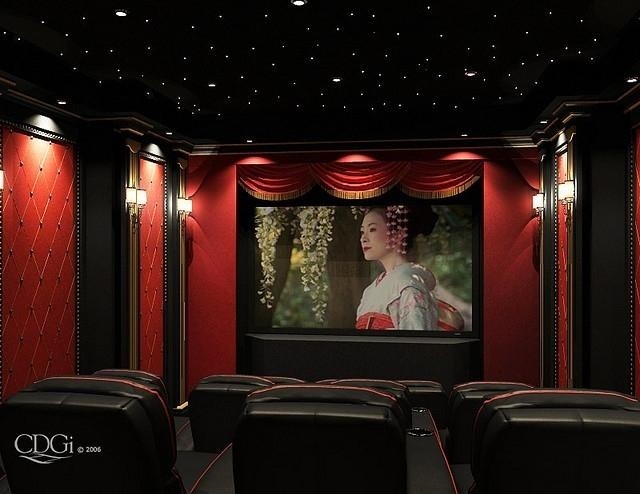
[
  {"left": 126, "top": 186, "right": 147, "bottom": 234},
  {"left": 532, "top": 180, "right": 575, "bottom": 232},
  {"left": 177, "top": 197, "right": 193, "bottom": 229}
]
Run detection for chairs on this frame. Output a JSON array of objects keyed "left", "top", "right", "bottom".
[{"left": 1, "top": 366, "right": 638, "bottom": 492}]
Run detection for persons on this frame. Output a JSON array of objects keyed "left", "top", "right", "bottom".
[{"left": 353, "top": 205, "right": 465, "bottom": 331}]
